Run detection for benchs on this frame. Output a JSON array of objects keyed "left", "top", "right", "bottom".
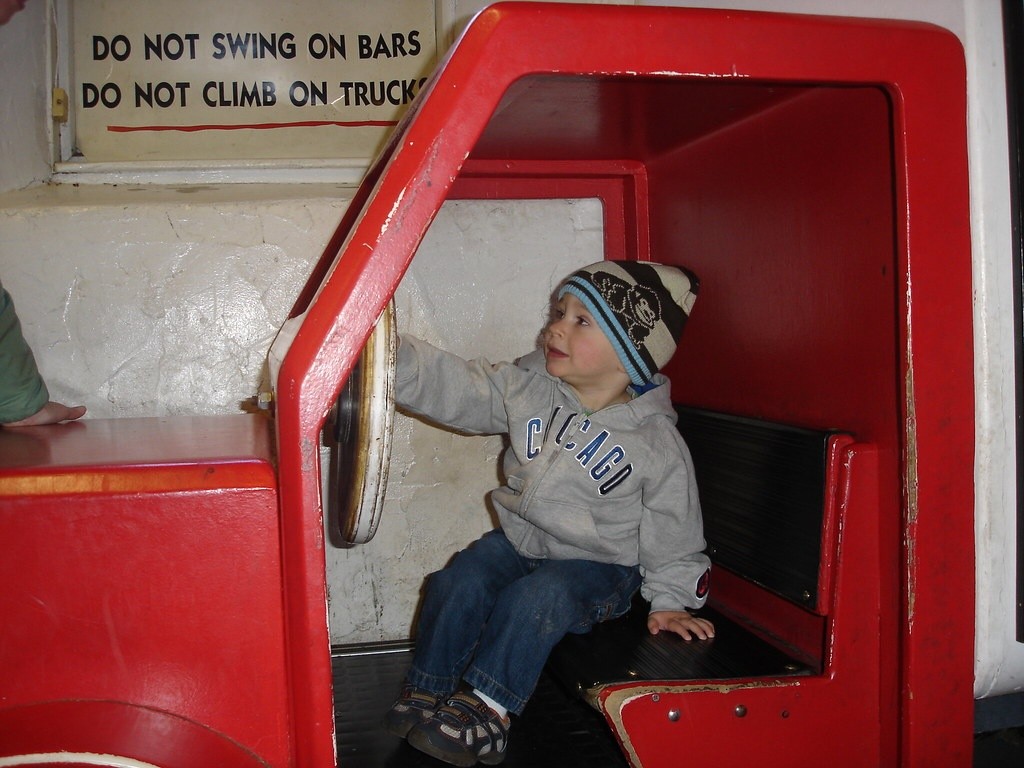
[{"left": 552, "top": 403, "right": 855, "bottom": 707}]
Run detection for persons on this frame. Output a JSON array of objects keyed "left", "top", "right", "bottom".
[
  {"left": 0, "top": 280, "right": 86, "bottom": 427},
  {"left": 382, "top": 261, "right": 716, "bottom": 767}
]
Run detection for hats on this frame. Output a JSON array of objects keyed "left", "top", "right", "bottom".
[{"left": 557, "top": 259, "right": 701, "bottom": 385}]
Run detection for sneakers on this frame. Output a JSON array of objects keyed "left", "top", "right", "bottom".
[
  {"left": 382, "top": 682, "right": 439, "bottom": 738},
  {"left": 407, "top": 690, "right": 509, "bottom": 767}
]
